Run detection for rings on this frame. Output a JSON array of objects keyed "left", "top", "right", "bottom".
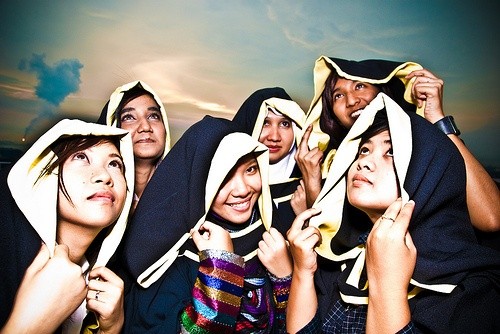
[
  {"left": 382, "top": 215, "right": 394, "bottom": 223},
  {"left": 95, "top": 292, "right": 99, "bottom": 300}
]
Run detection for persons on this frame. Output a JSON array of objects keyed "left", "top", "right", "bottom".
[
  {"left": 123, "top": 117, "right": 294, "bottom": 334},
  {"left": 293, "top": 55, "right": 500, "bottom": 231},
  {"left": 0, "top": 117, "right": 135, "bottom": 334},
  {"left": 96, "top": 79, "right": 170, "bottom": 202},
  {"left": 232, "top": 88, "right": 306, "bottom": 240},
  {"left": 286, "top": 92, "right": 500, "bottom": 334}
]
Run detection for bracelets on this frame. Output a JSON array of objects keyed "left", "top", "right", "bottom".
[{"left": 433, "top": 115, "right": 460, "bottom": 135}]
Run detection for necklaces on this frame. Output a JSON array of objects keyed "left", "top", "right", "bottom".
[{"left": 225, "top": 208, "right": 255, "bottom": 232}]
